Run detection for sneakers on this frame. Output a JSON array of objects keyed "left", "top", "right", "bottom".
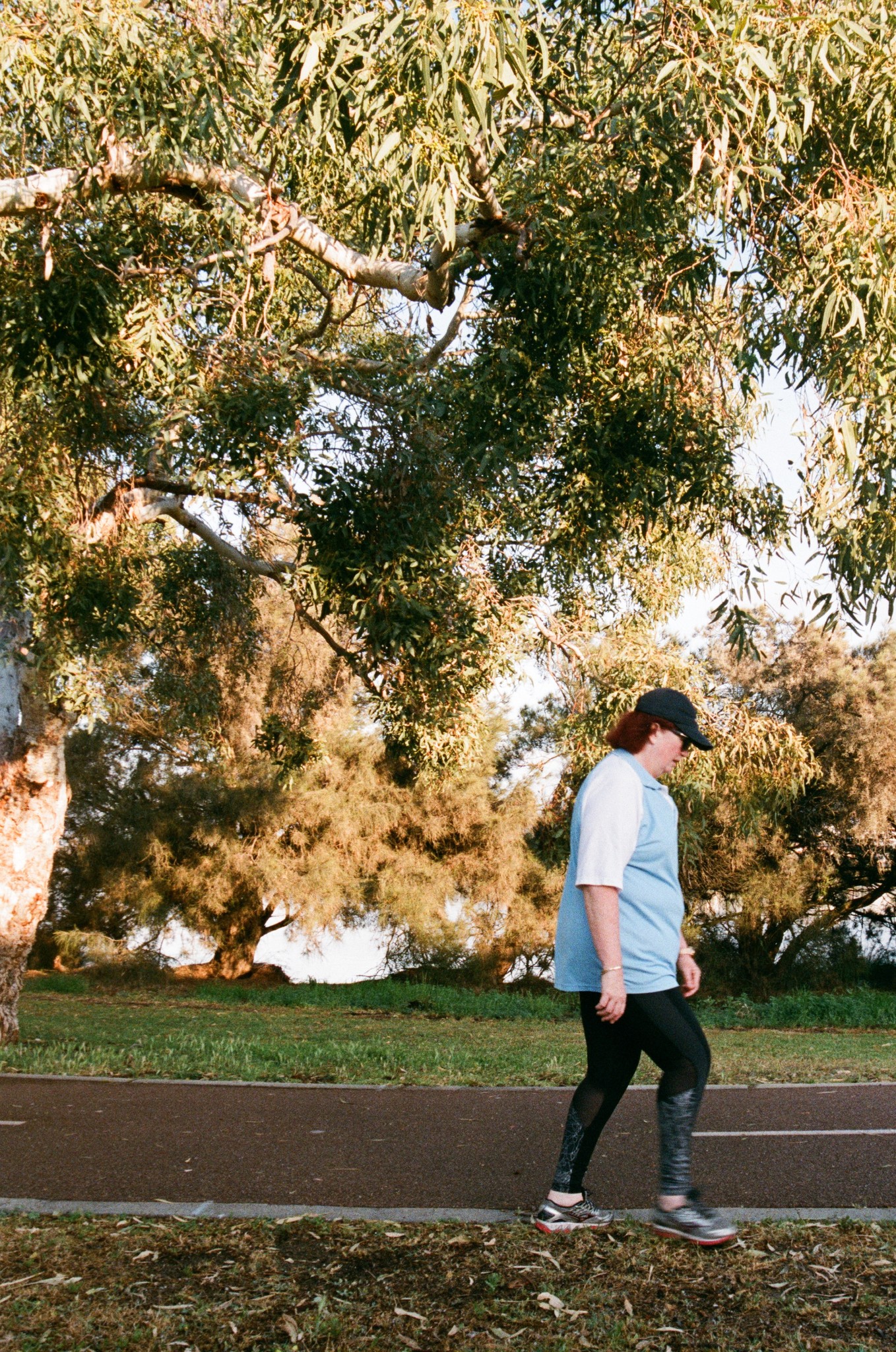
[
  {"left": 535, "top": 1189, "right": 613, "bottom": 1234},
  {"left": 650, "top": 1188, "right": 737, "bottom": 1244}
]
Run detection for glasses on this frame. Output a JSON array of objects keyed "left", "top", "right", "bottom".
[{"left": 659, "top": 725, "right": 693, "bottom": 752}]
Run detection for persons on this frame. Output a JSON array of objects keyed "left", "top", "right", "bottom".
[{"left": 535, "top": 687, "right": 738, "bottom": 1245}]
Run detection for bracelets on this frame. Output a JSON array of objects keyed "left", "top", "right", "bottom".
[
  {"left": 600, "top": 965, "right": 622, "bottom": 975},
  {"left": 679, "top": 946, "right": 696, "bottom": 955}
]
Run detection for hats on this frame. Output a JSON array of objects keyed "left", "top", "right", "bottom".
[{"left": 634, "top": 687, "right": 713, "bottom": 753}]
